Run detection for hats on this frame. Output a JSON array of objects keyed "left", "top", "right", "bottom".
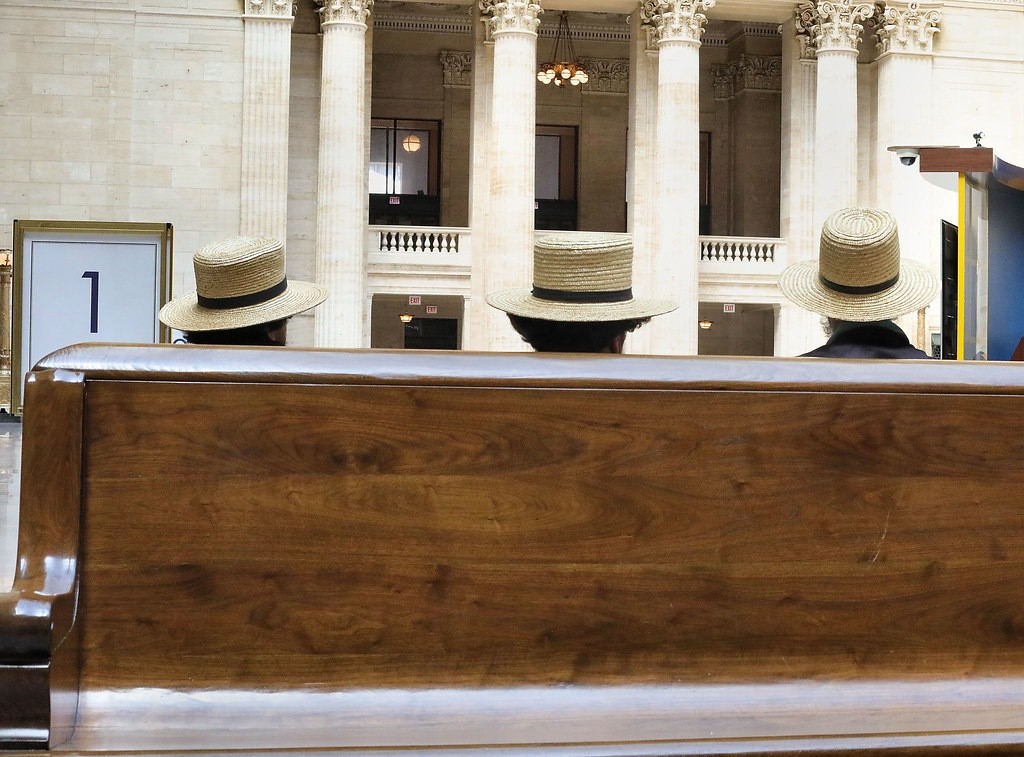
[
  {"left": 779, "top": 209, "right": 937, "bottom": 322},
  {"left": 157, "top": 231, "right": 329, "bottom": 332},
  {"left": 483, "top": 228, "right": 683, "bottom": 322}
]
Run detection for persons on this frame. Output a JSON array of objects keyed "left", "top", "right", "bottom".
[
  {"left": 777, "top": 206, "right": 943, "bottom": 359},
  {"left": 157, "top": 233, "right": 331, "bottom": 348},
  {"left": 484, "top": 227, "right": 678, "bottom": 354}
]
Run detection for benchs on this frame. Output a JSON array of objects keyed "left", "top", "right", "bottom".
[{"left": 0, "top": 344, "right": 1021, "bottom": 757}]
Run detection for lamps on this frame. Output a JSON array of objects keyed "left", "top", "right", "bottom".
[
  {"left": 538, "top": 11, "right": 590, "bottom": 87},
  {"left": 403, "top": 131, "right": 420, "bottom": 152},
  {"left": 699, "top": 301, "right": 714, "bottom": 329},
  {"left": 397, "top": 296, "right": 414, "bottom": 322}
]
